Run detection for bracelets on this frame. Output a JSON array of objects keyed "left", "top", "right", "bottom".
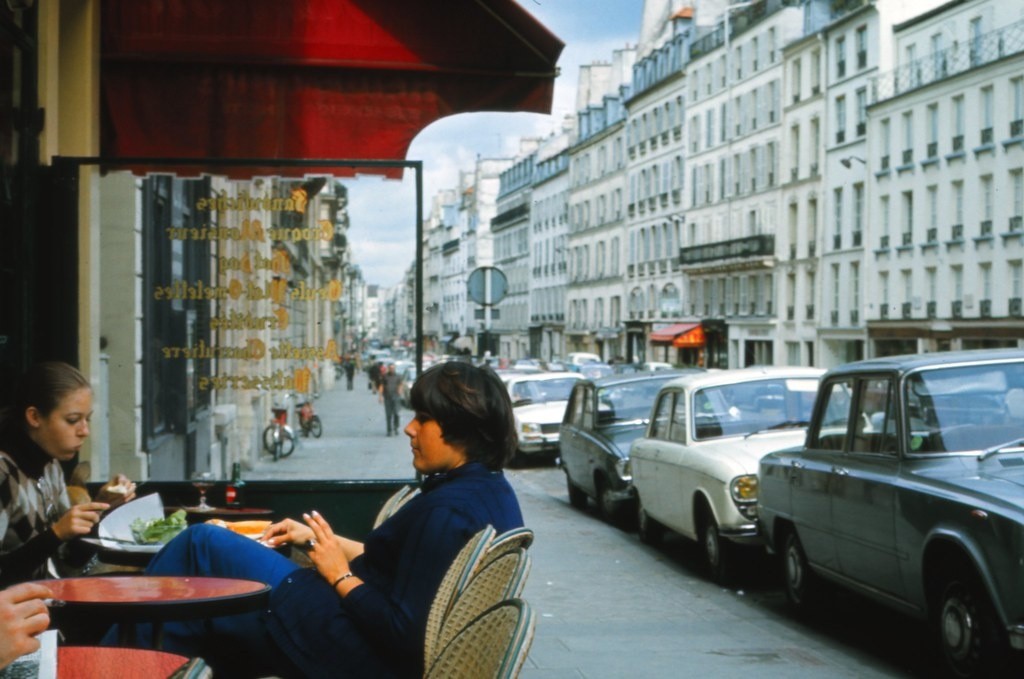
[{"left": 332, "top": 573, "right": 355, "bottom": 592}]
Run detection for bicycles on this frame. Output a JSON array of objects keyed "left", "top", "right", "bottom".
[
  {"left": 263, "top": 393, "right": 297, "bottom": 464},
  {"left": 293, "top": 393, "right": 323, "bottom": 439}
]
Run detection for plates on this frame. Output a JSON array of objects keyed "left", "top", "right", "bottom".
[{"left": 117, "top": 538, "right": 168, "bottom": 554}]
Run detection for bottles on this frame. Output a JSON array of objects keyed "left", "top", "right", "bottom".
[{"left": 224, "top": 462, "right": 246, "bottom": 509}]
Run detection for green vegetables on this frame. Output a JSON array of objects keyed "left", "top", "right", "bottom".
[{"left": 130, "top": 508, "right": 187, "bottom": 545}]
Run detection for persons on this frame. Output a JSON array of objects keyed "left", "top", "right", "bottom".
[
  {"left": 344, "top": 355, "right": 404, "bottom": 436},
  {"left": 101, "top": 361, "right": 524, "bottom": 679},
  {"left": 0, "top": 360, "right": 136, "bottom": 647},
  {"left": 0, "top": 583, "right": 53, "bottom": 669}
]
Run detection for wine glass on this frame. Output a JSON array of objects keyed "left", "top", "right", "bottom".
[{"left": 191, "top": 471, "right": 216, "bottom": 511}]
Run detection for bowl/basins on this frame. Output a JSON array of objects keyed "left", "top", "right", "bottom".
[{"left": 127, "top": 523, "right": 187, "bottom": 545}]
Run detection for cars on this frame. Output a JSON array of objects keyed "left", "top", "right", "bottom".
[
  {"left": 556, "top": 366, "right": 743, "bottom": 529},
  {"left": 505, "top": 370, "right": 615, "bottom": 454},
  {"left": 354, "top": 339, "right": 677, "bottom": 410},
  {"left": 628, "top": 364, "right": 876, "bottom": 587},
  {"left": 759, "top": 345, "right": 1024, "bottom": 679}
]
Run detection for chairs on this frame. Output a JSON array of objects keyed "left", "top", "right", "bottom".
[{"left": 373, "top": 485, "right": 535, "bottom": 679}]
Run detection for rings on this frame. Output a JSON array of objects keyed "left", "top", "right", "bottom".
[{"left": 305, "top": 539, "right": 315, "bottom": 550}]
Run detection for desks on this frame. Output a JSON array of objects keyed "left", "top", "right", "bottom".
[{"left": 7, "top": 503, "right": 294, "bottom": 679}]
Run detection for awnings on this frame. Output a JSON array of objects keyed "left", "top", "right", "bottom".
[
  {"left": 99, "top": 0, "right": 564, "bottom": 180},
  {"left": 648, "top": 323, "right": 705, "bottom": 347}
]
